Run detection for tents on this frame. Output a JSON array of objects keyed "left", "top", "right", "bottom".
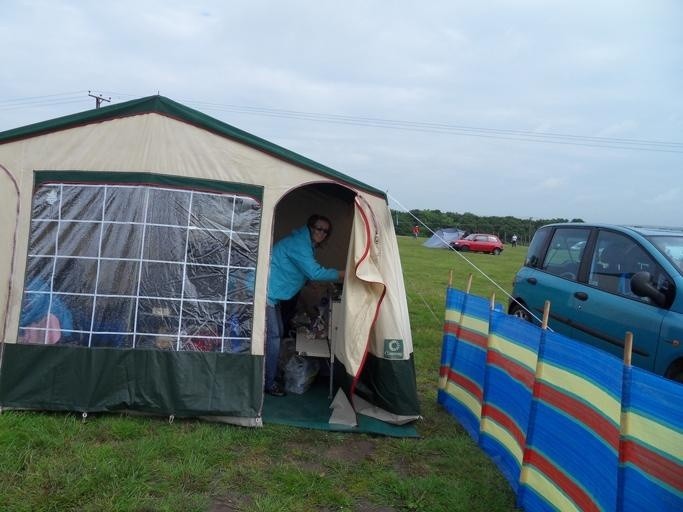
[
  {"left": 0, "top": 93, "right": 424, "bottom": 439},
  {"left": 422, "top": 227, "right": 471, "bottom": 252}
]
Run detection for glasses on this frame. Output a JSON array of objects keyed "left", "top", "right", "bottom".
[{"left": 316, "top": 227, "right": 328, "bottom": 234}]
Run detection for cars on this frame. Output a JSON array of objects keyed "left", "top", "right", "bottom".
[
  {"left": 506, "top": 218, "right": 682, "bottom": 384},
  {"left": 450, "top": 232, "right": 503, "bottom": 256}
]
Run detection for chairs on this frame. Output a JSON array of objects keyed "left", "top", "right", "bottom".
[{"left": 597, "top": 244, "right": 625, "bottom": 292}]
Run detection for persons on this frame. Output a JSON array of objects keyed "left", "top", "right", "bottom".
[
  {"left": 243, "top": 212, "right": 345, "bottom": 397},
  {"left": 413, "top": 224, "right": 420, "bottom": 238},
  {"left": 510, "top": 233, "right": 518, "bottom": 247}
]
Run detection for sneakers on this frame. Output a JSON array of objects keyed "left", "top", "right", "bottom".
[{"left": 265, "top": 384, "right": 286, "bottom": 397}]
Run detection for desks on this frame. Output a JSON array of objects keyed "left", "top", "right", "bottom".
[{"left": 325, "top": 283, "right": 342, "bottom": 400}]
